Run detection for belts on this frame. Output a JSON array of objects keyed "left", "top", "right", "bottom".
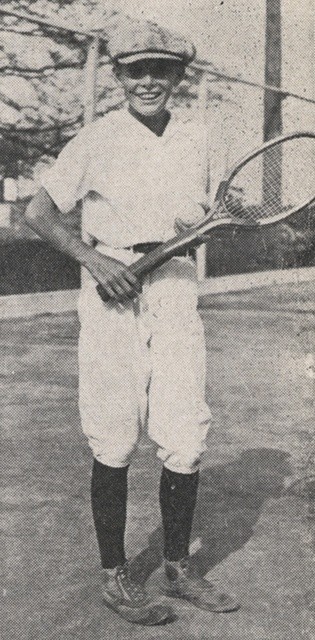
[{"left": 124, "top": 242, "right": 197, "bottom": 261}]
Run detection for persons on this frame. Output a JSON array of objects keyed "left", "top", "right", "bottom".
[{"left": 22, "top": 17, "right": 241, "bottom": 627}]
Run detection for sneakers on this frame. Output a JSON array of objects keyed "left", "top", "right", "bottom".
[
  {"left": 98, "top": 557, "right": 171, "bottom": 626},
  {"left": 158, "top": 552, "right": 239, "bottom": 612}
]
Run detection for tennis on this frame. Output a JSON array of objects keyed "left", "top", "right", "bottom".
[{"left": 176, "top": 202, "right": 206, "bottom": 229}]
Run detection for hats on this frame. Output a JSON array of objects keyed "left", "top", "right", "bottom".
[{"left": 106, "top": 20, "right": 196, "bottom": 66}]
